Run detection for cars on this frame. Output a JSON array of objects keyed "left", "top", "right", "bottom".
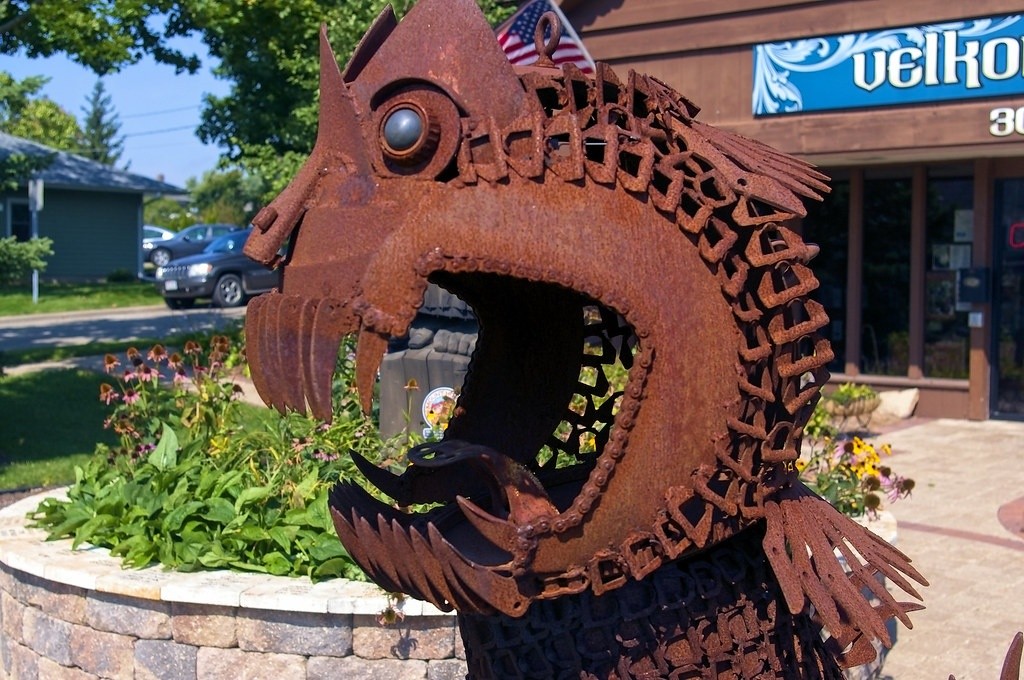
[
  {"left": 142, "top": 224, "right": 241, "bottom": 269},
  {"left": 141, "top": 226, "right": 180, "bottom": 242},
  {"left": 155, "top": 229, "right": 287, "bottom": 309}
]
